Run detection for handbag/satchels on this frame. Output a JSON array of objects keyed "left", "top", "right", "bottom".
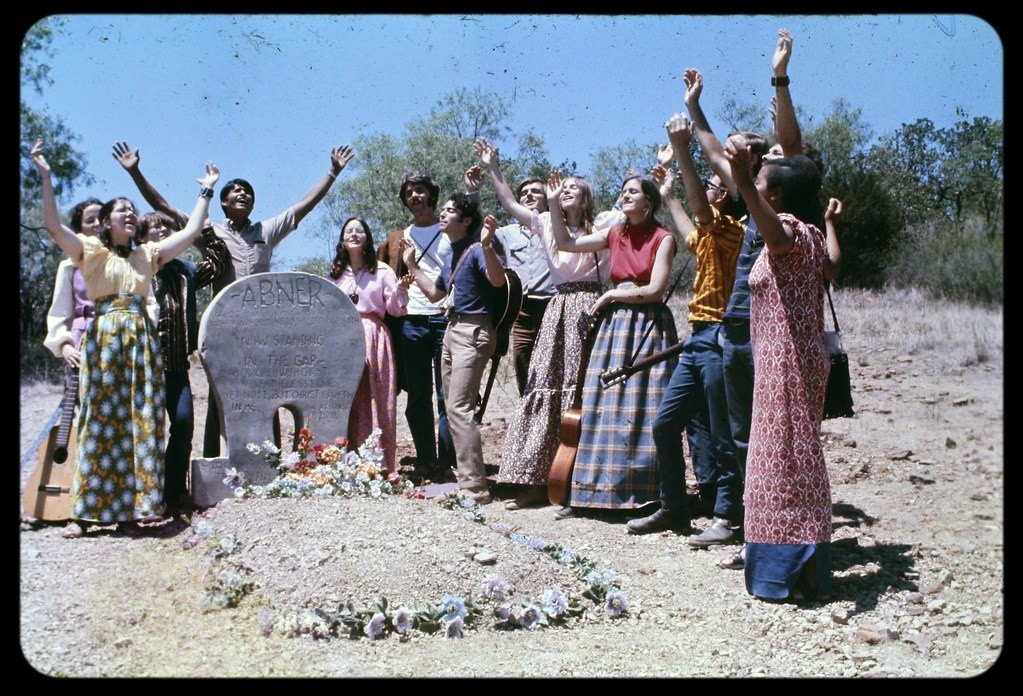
[{"left": 822, "top": 331, "right": 855, "bottom": 419}]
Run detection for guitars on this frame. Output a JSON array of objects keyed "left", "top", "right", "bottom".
[
  {"left": 22, "top": 337, "right": 84, "bottom": 522},
  {"left": 596, "top": 340, "right": 685, "bottom": 391},
  {"left": 472, "top": 265, "right": 523, "bottom": 424}
]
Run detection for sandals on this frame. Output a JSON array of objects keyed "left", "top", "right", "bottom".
[{"left": 719, "top": 544, "right": 746, "bottom": 569}]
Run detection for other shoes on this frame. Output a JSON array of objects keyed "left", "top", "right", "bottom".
[
  {"left": 62, "top": 522, "right": 85, "bottom": 538},
  {"left": 118, "top": 519, "right": 144, "bottom": 537},
  {"left": 555, "top": 507, "right": 592, "bottom": 518},
  {"left": 506, "top": 492, "right": 540, "bottom": 509},
  {"left": 386, "top": 468, "right": 400, "bottom": 484},
  {"left": 435, "top": 487, "right": 491, "bottom": 503}
]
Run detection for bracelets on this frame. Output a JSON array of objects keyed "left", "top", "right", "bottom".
[
  {"left": 199, "top": 188, "right": 214, "bottom": 199},
  {"left": 769, "top": 74, "right": 791, "bottom": 86},
  {"left": 328, "top": 169, "right": 338, "bottom": 179}
]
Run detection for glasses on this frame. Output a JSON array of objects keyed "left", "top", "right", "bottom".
[
  {"left": 703, "top": 179, "right": 729, "bottom": 191},
  {"left": 110, "top": 205, "right": 140, "bottom": 216}
]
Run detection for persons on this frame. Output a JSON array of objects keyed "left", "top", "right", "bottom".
[{"left": 31, "top": 28, "right": 846, "bottom": 601}]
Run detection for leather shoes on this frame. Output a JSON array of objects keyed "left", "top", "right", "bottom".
[
  {"left": 627, "top": 509, "right": 691, "bottom": 533},
  {"left": 688, "top": 517, "right": 740, "bottom": 545}
]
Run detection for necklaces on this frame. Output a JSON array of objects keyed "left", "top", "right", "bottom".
[{"left": 345, "top": 267, "right": 367, "bottom": 304}]
